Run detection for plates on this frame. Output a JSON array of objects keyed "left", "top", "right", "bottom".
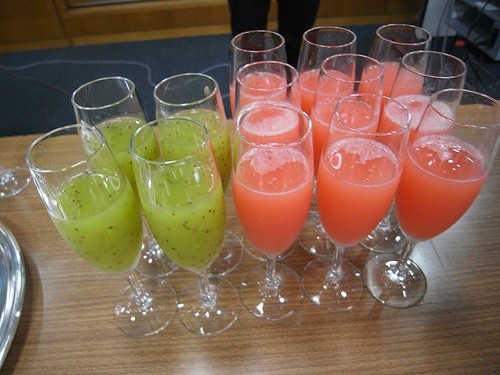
[{"left": 0, "top": 223, "right": 26, "bottom": 370}]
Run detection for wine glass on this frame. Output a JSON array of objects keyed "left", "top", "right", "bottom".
[
  {"left": 301, "top": 93, "right": 412, "bottom": 314},
  {"left": 26, "top": 125, "right": 177, "bottom": 337},
  {"left": 71, "top": 77, "right": 179, "bottom": 279},
  {"left": 239, "top": 61, "right": 303, "bottom": 258},
  {"left": 0, "top": 156, "right": 32, "bottom": 199},
  {"left": 291, "top": 25, "right": 357, "bottom": 119},
  {"left": 357, "top": 24, "right": 433, "bottom": 106},
  {"left": 129, "top": 117, "right": 239, "bottom": 335},
  {"left": 360, "top": 50, "right": 466, "bottom": 252},
  {"left": 298, "top": 54, "right": 384, "bottom": 256},
  {"left": 231, "top": 101, "right": 314, "bottom": 320},
  {"left": 154, "top": 74, "right": 242, "bottom": 277},
  {"left": 230, "top": 30, "right": 288, "bottom": 127},
  {"left": 363, "top": 88, "right": 500, "bottom": 308}
]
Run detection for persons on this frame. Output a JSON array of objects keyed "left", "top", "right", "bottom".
[{"left": 227, "top": 0, "right": 320, "bottom": 91}]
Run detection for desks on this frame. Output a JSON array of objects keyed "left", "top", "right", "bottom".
[{"left": 0, "top": 104, "right": 500, "bottom": 375}]
